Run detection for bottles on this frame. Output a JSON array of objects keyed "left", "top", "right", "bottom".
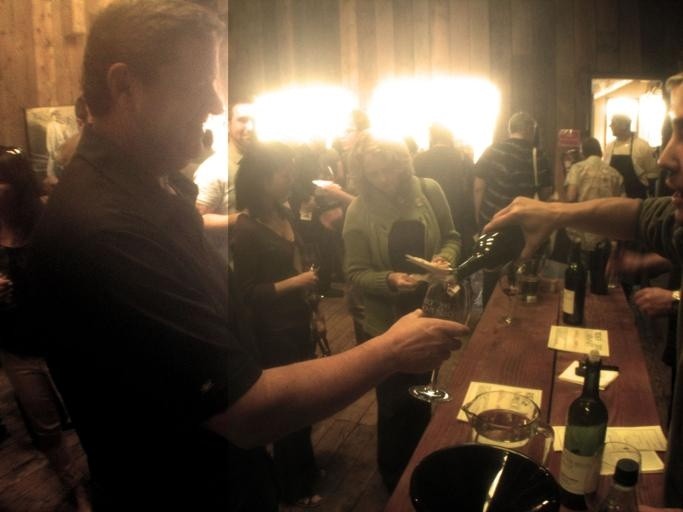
[
  {"left": 589, "top": 240, "right": 610, "bottom": 295},
  {"left": 454, "top": 226, "right": 524, "bottom": 281},
  {"left": 562, "top": 238, "right": 585, "bottom": 326},
  {"left": 595, "top": 458, "right": 639, "bottom": 512},
  {"left": 558, "top": 360, "right": 609, "bottom": 511}
]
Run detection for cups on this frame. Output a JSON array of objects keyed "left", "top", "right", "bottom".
[
  {"left": 523, "top": 257, "right": 546, "bottom": 306},
  {"left": 463, "top": 391, "right": 555, "bottom": 469}
]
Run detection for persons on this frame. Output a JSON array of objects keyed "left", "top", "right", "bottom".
[
  {"left": 340, "top": 127, "right": 463, "bottom": 496},
  {"left": 482, "top": 72, "right": 683, "bottom": 508},
  {"left": 32, "top": 0, "right": 471, "bottom": 511},
  {"left": 602, "top": 113, "right": 660, "bottom": 200},
  {"left": 472, "top": 111, "right": 556, "bottom": 263},
  {"left": 190, "top": 96, "right": 267, "bottom": 267},
  {"left": 62, "top": 97, "right": 88, "bottom": 170},
  {"left": 311, "top": 158, "right": 357, "bottom": 210},
  {"left": 42, "top": 111, "right": 69, "bottom": 177},
  {"left": 414, "top": 124, "right": 474, "bottom": 232},
  {"left": 561, "top": 138, "right": 626, "bottom": 272},
  {"left": 229, "top": 144, "right": 333, "bottom": 365},
  {"left": 0, "top": 144, "right": 47, "bottom": 362},
  {"left": 290, "top": 143, "right": 346, "bottom": 299}
]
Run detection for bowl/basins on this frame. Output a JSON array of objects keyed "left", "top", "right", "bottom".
[{"left": 409, "top": 441, "right": 559, "bottom": 512}]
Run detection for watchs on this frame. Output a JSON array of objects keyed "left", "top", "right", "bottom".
[{"left": 671, "top": 289, "right": 682, "bottom": 309}]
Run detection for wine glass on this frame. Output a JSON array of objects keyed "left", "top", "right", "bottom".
[
  {"left": 302, "top": 253, "right": 325, "bottom": 302},
  {"left": 585, "top": 441, "right": 641, "bottom": 512},
  {"left": 408, "top": 276, "right": 466, "bottom": 404},
  {"left": 495, "top": 265, "right": 522, "bottom": 327}
]
